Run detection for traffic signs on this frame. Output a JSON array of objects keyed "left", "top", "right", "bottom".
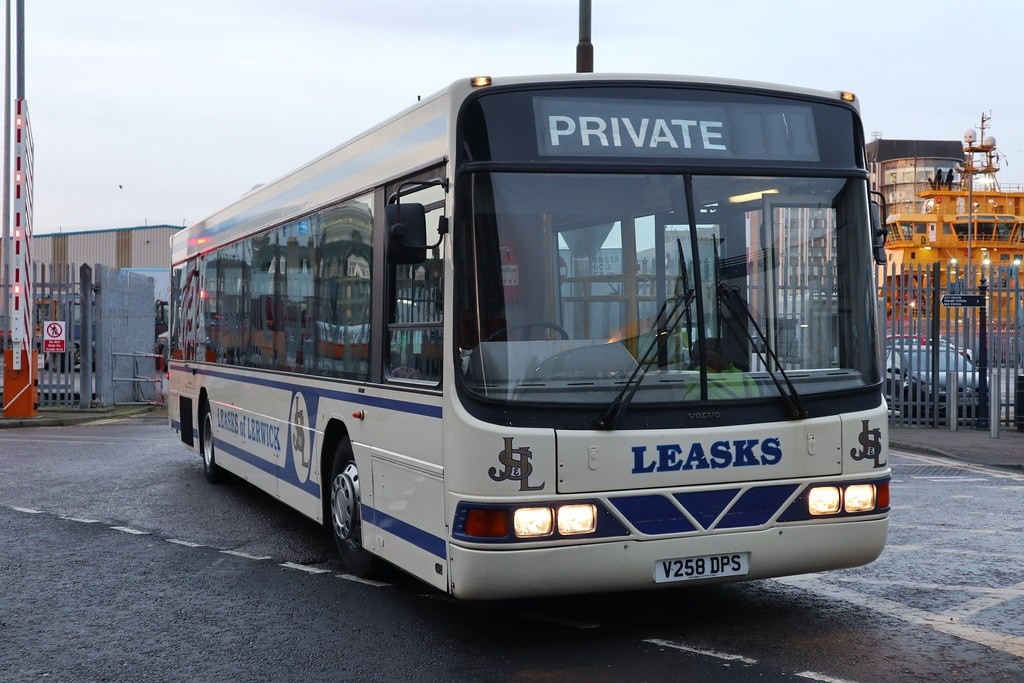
[{"left": 941, "top": 294, "right": 987, "bottom": 308}]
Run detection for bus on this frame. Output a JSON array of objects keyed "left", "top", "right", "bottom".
[{"left": 165, "top": 70, "right": 892, "bottom": 602}]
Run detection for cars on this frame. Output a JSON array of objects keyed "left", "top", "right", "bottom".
[
  {"left": 886, "top": 334, "right": 974, "bottom": 362},
  {"left": 886, "top": 346, "right": 993, "bottom": 427}
]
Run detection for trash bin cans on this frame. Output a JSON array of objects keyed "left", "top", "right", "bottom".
[{"left": 1014, "top": 375, "right": 1024, "bottom": 431}]
